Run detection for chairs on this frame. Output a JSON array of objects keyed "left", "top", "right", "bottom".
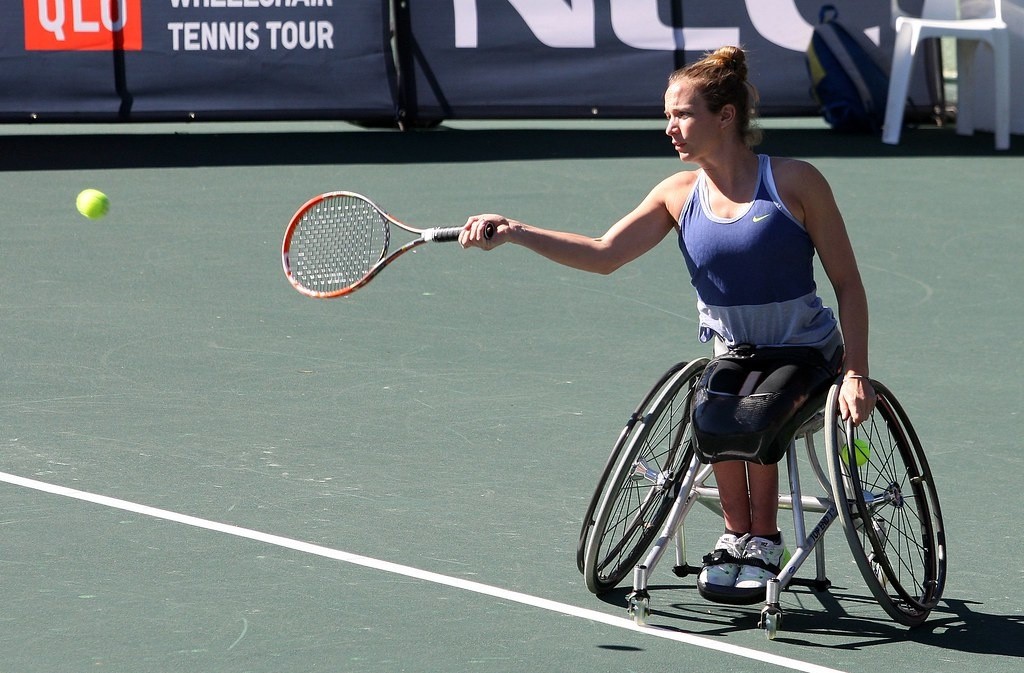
[{"left": 881, "top": 1, "right": 1010, "bottom": 150}]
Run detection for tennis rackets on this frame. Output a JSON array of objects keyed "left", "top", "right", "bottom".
[{"left": 281, "top": 191, "right": 497, "bottom": 301}]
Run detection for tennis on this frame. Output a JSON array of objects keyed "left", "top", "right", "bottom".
[
  {"left": 74, "top": 188, "right": 113, "bottom": 221},
  {"left": 840, "top": 439, "right": 871, "bottom": 468}
]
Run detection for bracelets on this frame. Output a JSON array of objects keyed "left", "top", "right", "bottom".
[{"left": 844, "top": 374, "right": 871, "bottom": 381}]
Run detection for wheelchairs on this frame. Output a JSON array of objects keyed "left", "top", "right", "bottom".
[{"left": 575, "top": 350, "right": 949, "bottom": 641}]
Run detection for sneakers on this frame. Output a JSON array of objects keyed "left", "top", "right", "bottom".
[
  {"left": 735, "top": 526, "right": 791, "bottom": 588},
  {"left": 699, "top": 532, "right": 751, "bottom": 586}
]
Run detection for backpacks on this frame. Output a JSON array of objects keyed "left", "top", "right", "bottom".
[{"left": 805, "top": 3, "right": 920, "bottom": 136}]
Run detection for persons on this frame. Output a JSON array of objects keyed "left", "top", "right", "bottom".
[{"left": 459, "top": 47, "right": 877, "bottom": 603}]
